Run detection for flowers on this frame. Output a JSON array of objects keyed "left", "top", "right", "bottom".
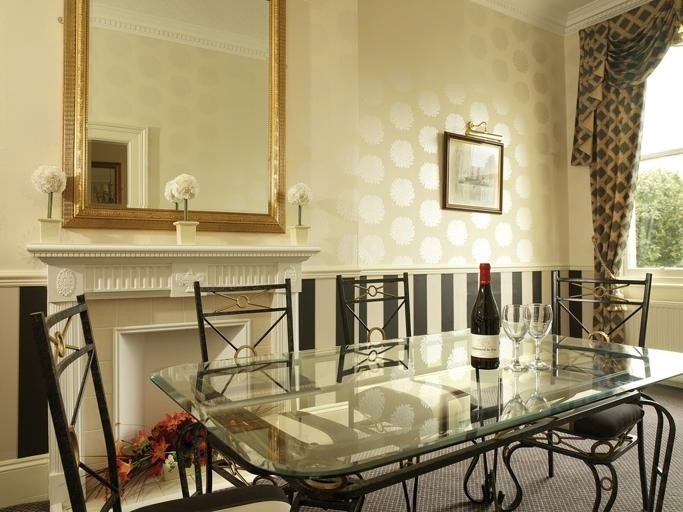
[
  {"left": 81, "top": 411, "right": 218, "bottom": 499},
  {"left": 165, "top": 180, "right": 183, "bottom": 210},
  {"left": 32, "top": 166, "right": 68, "bottom": 217},
  {"left": 173, "top": 173, "right": 198, "bottom": 220},
  {"left": 286, "top": 182, "right": 313, "bottom": 226}
]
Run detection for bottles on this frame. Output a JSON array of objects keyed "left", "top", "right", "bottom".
[{"left": 469, "top": 262, "right": 502, "bottom": 370}]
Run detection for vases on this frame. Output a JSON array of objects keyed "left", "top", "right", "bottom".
[
  {"left": 160, "top": 457, "right": 188, "bottom": 479},
  {"left": 38, "top": 216, "right": 63, "bottom": 243},
  {"left": 289, "top": 225, "right": 311, "bottom": 247},
  {"left": 173, "top": 220, "right": 200, "bottom": 246}
]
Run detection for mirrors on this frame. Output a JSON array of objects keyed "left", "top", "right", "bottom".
[
  {"left": 87, "top": 120, "right": 148, "bottom": 209},
  {"left": 61, "top": 1, "right": 287, "bottom": 235}
]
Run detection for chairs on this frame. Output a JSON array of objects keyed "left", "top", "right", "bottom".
[
  {"left": 497, "top": 272, "right": 651, "bottom": 511},
  {"left": 28, "top": 294, "right": 292, "bottom": 512},
  {"left": 340, "top": 276, "right": 490, "bottom": 511},
  {"left": 189, "top": 279, "right": 295, "bottom": 494}
]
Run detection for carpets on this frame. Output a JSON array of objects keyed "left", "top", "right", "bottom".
[{"left": 258, "top": 385, "right": 683, "bottom": 512}]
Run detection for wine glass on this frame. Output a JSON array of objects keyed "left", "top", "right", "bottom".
[
  {"left": 502, "top": 304, "right": 553, "bottom": 370},
  {"left": 499, "top": 371, "right": 551, "bottom": 422}
]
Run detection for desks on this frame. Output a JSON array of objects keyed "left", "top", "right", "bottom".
[{"left": 144, "top": 327, "right": 683, "bottom": 512}]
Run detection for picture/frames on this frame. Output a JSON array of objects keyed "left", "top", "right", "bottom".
[
  {"left": 91, "top": 161, "right": 122, "bottom": 204},
  {"left": 441, "top": 130, "right": 505, "bottom": 214}
]
